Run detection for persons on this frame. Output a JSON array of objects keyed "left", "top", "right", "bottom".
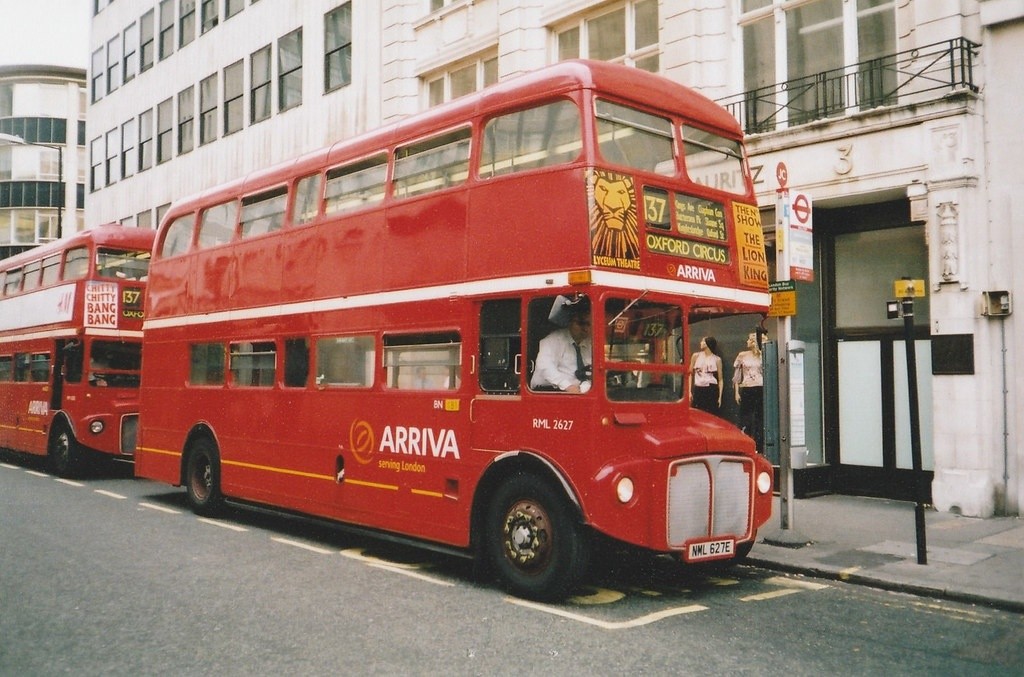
[
  {"left": 414, "top": 366, "right": 433, "bottom": 391},
  {"left": 530, "top": 308, "right": 593, "bottom": 393},
  {"left": 688, "top": 332, "right": 764, "bottom": 452}
]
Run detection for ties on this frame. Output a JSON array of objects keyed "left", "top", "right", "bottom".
[{"left": 573, "top": 343, "right": 586, "bottom": 381}]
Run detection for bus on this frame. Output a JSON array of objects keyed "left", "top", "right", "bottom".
[
  {"left": 131, "top": 59, "right": 774, "bottom": 604},
  {"left": 0, "top": 225, "right": 160, "bottom": 479}
]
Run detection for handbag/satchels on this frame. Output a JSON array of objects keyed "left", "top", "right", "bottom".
[{"left": 733, "top": 360, "right": 742, "bottom": 384}]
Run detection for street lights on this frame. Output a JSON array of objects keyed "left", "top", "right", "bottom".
[{"left": 0, "top": 133, "right": 64, "bottom": 238}]
